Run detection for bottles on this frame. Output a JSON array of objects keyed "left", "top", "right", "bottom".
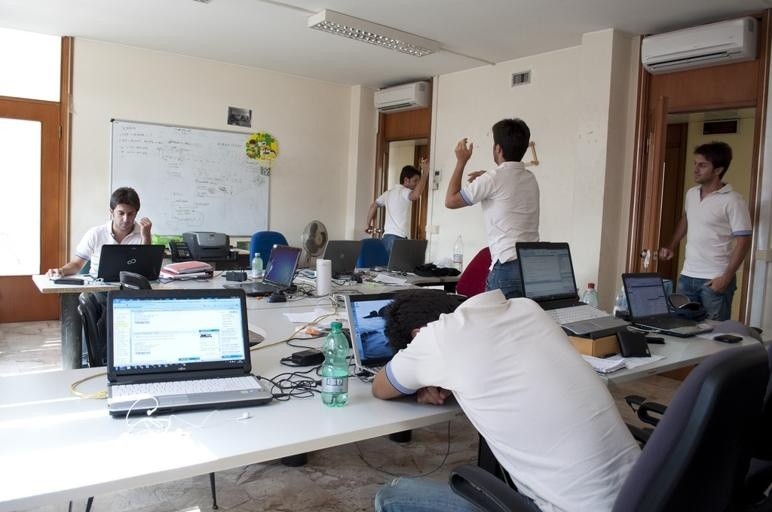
[
  {"left": 581, "top": 282, "right": 597, "bottom": 311},
  {"left": 321, "top": 321, "right": 349, "bottom": 408},
  {"left": 452, "top": 235, "right": 464, "bottom": 275},
  {"left": 252, "top": 252, "right": 263, "bottom": 285}
]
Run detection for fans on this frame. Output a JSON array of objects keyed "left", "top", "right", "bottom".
[{"left": 297, "top": 220, "right": 329, "bottom": 268}]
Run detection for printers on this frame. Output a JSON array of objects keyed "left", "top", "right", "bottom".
[{"left": 168, "top": 231, "right": 233, "bottom": 266}]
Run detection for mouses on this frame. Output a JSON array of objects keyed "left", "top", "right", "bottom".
[
  {"left": 269, "top": 294, "right": 288, "bottom": 304},
  {"left": 50, "top": 269, "right": 65, "bottom": 280}
]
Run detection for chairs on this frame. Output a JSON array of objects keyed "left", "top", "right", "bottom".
[
  {"left": 249, "top": 231, "right": 288, "bottom": 270},
  {"left": 446, "top": 341, "right": 772, "bottom": 512},
  {"left": 355, "top": 239, "right": 389, "bottom": 269},
  {"left": 625, "top": 395, "right": 667, "bottom": 427}
]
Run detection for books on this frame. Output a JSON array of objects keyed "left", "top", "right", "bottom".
[
  {"left": 581, "top": 354, "right": 626, "bottom": 374},
  {"left": 159, "top": 259, "right": 214, "bottom": 284}
]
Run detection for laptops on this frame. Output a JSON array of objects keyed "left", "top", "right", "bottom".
[
  {"left": 514, "top": 241, "right": 630, "bottom": 337},
  {"left": 387, "top": 240, "right": 428, "bottom": 270},
  {"left": 344, "top": 292, "right": 461, "bottom": 377},
  {"left": 99, "top": 243, "right": 162, "bottom": 285},
  {"left": 106, "top": 290, "right": 273, "bottom": 417},
  {"left": 621, "top": 271, "right": 716, "bottom": 337},
  {"left": 322, "top": 239, "right": 363, "bottom": 274},
  {"left": 221, "top": 244, "right": 302, "bottom": 297}
]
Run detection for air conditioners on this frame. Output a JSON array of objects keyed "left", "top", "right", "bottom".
[
  {"left": 373, "top": 80, "right": 430, "bottom": 114},
  {"left": 641, "top": 15, "right": 757, "bottom": 76}
]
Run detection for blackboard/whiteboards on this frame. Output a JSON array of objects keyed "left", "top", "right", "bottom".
[{"left": 110, "top": 117, "right": 273, "bottom": 239}]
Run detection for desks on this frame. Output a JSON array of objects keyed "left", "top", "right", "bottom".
[
  {"left": 0, "top": 304, "right": 687, "bottom": 512},
  {"left": 333, "top": 272, "right": 771, "bottom": 390},
  {"left": 0, "top": 272, "right": 566, "bottom": 372}
]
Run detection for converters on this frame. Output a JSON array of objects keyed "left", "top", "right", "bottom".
[
  {"left": 291, "top": 348, "right": 326, "bottom": 367},
  {"left": 221, "top": 270, "right": 248, "bottom": 282}
]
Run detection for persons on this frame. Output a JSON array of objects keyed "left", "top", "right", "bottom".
[
  {"left": 657, "top": 140, "right": 752, "bottom": 322},
  {"left": 372, "top": 288, "right": 642, "bottom": 512},
  {"left": 45, "top": 187, "right": 151, "bottom": 366},
  {"left": 363, "top": 157, "right": 430, "bottom": 256},
  {"left": 444, "top": 118, "right": 540, "bottom": 301}
]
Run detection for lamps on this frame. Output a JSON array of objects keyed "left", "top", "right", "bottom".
[{"left": 307, "top": 8, "right": 446, "bottom": 58}]
